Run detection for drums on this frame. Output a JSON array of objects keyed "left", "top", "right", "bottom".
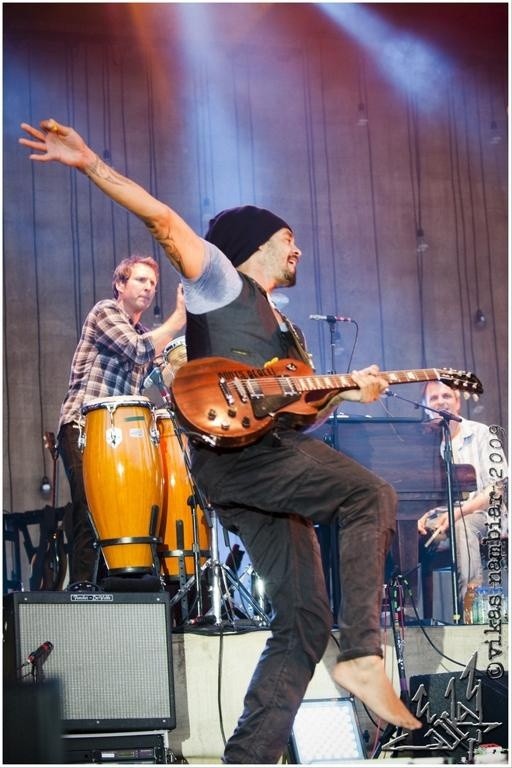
[
  {"left": 153, "top": 403, "right": 212, "bottom": 582},
  {"left": 81, "top": 395, "right": 165, "bottom": 579}
]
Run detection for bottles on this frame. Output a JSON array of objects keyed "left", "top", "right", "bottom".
[
  {"left": 380, "top": 584, "right": 392, "bottom": 628},
  {"left": 463, "top": 584, "right": 508, "bottom": 625}
]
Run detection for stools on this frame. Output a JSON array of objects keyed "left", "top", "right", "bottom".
[{"left": 421, "top": 537, "right": 509, "bottom": 624}]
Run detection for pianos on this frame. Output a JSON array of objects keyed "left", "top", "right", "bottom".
[{"left": 303, "top": 414, "right": 478, "bottom": 626}]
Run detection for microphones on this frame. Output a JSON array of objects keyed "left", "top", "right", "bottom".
[
  {"left": 309, "top": 315, "right": 352, "bottom": 322},
  {"left": 143, "top": 367, "right": 161, "bottom": 388}
]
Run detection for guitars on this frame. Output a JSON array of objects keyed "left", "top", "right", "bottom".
[
  {"left": 27, "top": 431, "right": 71, "bottom": 594},
  {"left": 168, "top": 359, "right": 483, "bottom": 447}
]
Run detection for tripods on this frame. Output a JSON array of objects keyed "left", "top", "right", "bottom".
[{"left": 169, "top": 505, "right": 271, "bottom": 629}]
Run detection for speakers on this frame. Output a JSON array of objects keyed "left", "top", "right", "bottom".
[
  {"left": 390, "top": 668, "right": 507, "bottom": 758},
  {"left": 9, "top": 591, "right": 176, "bottom": 735}
]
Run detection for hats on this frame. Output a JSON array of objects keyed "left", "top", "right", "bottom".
[{"left": 202, "top": 203, "right": 295, "bottom": 270}]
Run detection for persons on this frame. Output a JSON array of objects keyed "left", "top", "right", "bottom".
[
  {"left": 18, "top": 115, "right": 425, "bottom": 764},
  {"left": 55, "top": 256, "right": 190, "bottom": 594},
  {"left": 416, "top": 379, "right": 508, "bottom": 623}
]
